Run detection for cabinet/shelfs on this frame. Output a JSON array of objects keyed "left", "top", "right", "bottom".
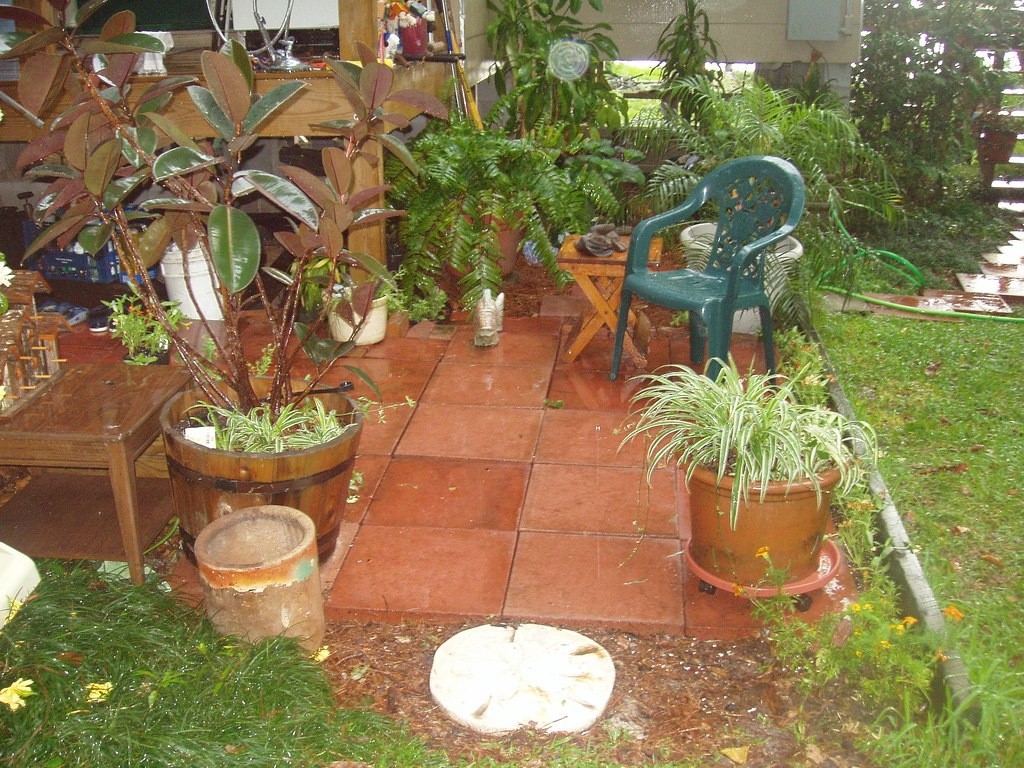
[{"left": 0, "top": 0, "right": 454, "bottom": 280}]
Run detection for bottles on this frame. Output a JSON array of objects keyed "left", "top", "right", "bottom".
[{"left": 473, "top": 289, "right": 500, "bottom": 346}]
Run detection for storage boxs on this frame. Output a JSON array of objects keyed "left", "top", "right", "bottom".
[{"left": 22, "top": 208, "right": 157, "bottom": 287}]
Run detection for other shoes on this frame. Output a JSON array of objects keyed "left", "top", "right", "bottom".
[{"left": 85, "top": 304, "right": 110, "bottom": 332}]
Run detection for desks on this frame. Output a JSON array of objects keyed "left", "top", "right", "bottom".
[
  {"left": 0, "top": 363, "right": 196, "bottom": 585},
  {"left": 556, "top": 234, "right": 662, "bottom": 369}
]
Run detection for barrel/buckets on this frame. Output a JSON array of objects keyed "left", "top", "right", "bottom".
[
  {"left": 679, "top": 222, "right": 803, "bottom": 337},
  {"left": 159, "top": 241, "right": 224, "bottom": 320}
]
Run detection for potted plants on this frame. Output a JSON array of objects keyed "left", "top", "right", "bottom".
[
  {"left": 642, "top": 72, "right": 910, "bottom": 336},
  {"left": 978, "top": 114, "right": 1024, "bottom": 166},
  {"left": 317, "top": 259, "right": 410, "bottom": 347},
  {"left": 99, "top": 293, "right": 191, "bottom": 365},
  {"left": 381, "top": 83, "right": 641, "bottom": 319},
  {"left": 615, "top": 352, "right": 879, "bottom": 612},
  {"left": 0, "top": 0, "right": 447, "bottom": 566}
]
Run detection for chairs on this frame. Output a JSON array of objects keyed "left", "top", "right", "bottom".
[{"left": 610, "top": 155, "right": 807, "bottom": 386}]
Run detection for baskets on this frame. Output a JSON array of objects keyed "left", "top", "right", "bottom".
[{"left": 22, "top": 222, "right": 122, "bottom": 284}]
